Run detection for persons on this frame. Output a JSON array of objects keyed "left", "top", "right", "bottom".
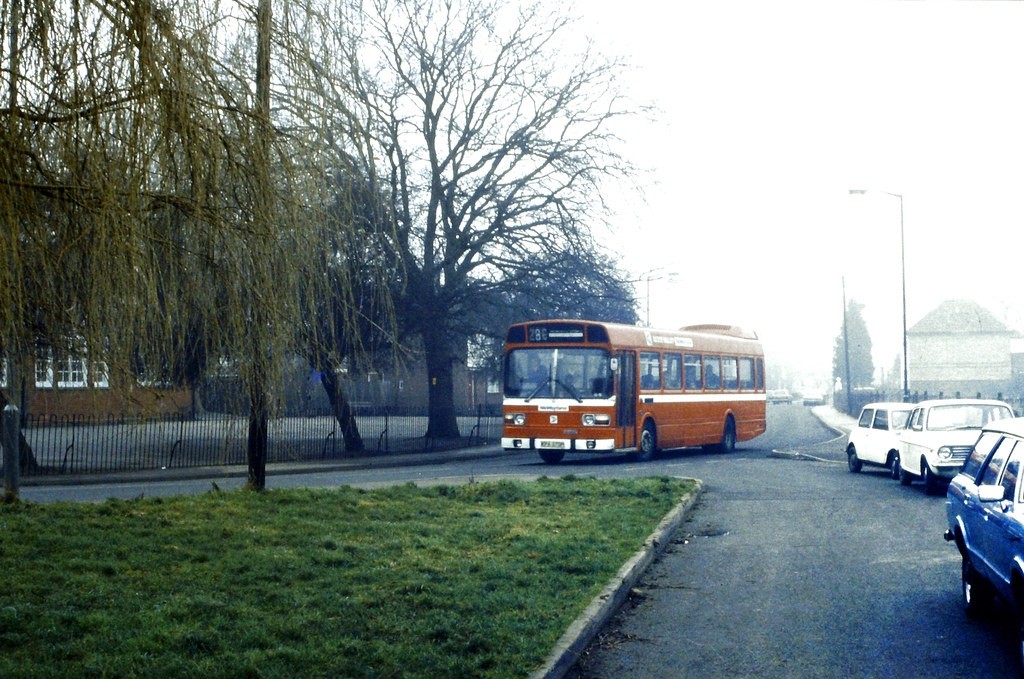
[
  {"left": 663, "top": 371, "right": 681, "bottom": 390},
  {"left": 642, "top": 374, "right": 655, "bottom": 390},
  {"left": 563, "top": 373, "right": 575, "bottom": 398},
  {"left": 528, "top": 356, "right": 548, "bottom": 384},
  {"left": 705, "top": 365, "right": 718, "bottom": 387}
]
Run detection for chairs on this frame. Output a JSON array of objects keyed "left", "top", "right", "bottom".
[{"left": 591, "top": 378, "right": 604, "bottom": 397}]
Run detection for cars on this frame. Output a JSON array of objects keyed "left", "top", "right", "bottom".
[
  {"left": 898, "top": 398, "right": 1017, "bottom": 494},
  {"left": 944, "top": 417, "right": 1024, "bottom": 637},
  {"left": 771, "top": 389, "right": 794, "bottom": 405},
  {"left": 846, "top": 402, "right": 915, "bottom": 480},
  {"left": 803, "top": 390, "right": 826, "bottom": 406}
]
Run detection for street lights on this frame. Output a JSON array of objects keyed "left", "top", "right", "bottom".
[{"left": 848, "top": 187, "right": 911, "bottom": 401}]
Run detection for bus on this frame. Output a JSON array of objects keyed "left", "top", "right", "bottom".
[{"left": 495, "top": 319, "right": 767, "bottom": 464}]
